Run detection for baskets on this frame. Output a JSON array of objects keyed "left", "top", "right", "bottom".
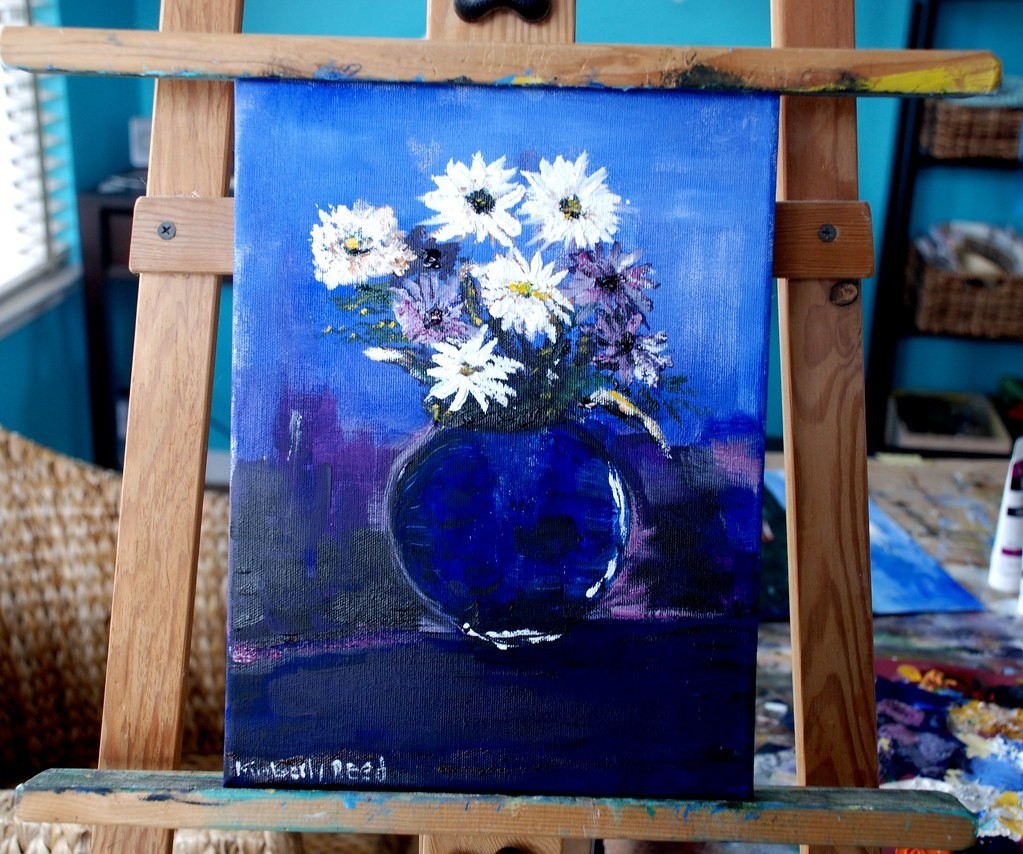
[
  {"left": 897, "top": 241, "right": 1023, "bottom": 340},
  {"left": 915, "top": 96, "right": 1023, "bottom": 158}
]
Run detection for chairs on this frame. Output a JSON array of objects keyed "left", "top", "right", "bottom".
[{"left": 0, "top": 426, "right": 405, "bottom": 854}]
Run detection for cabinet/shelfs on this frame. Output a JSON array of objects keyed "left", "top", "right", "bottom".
[{"left": 866, "top": 0, "right": 1023, "bottom": 458}]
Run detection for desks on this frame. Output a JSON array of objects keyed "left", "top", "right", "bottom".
[{"left": 79, "top": 174, "right": 149, "bottom": 474}]
